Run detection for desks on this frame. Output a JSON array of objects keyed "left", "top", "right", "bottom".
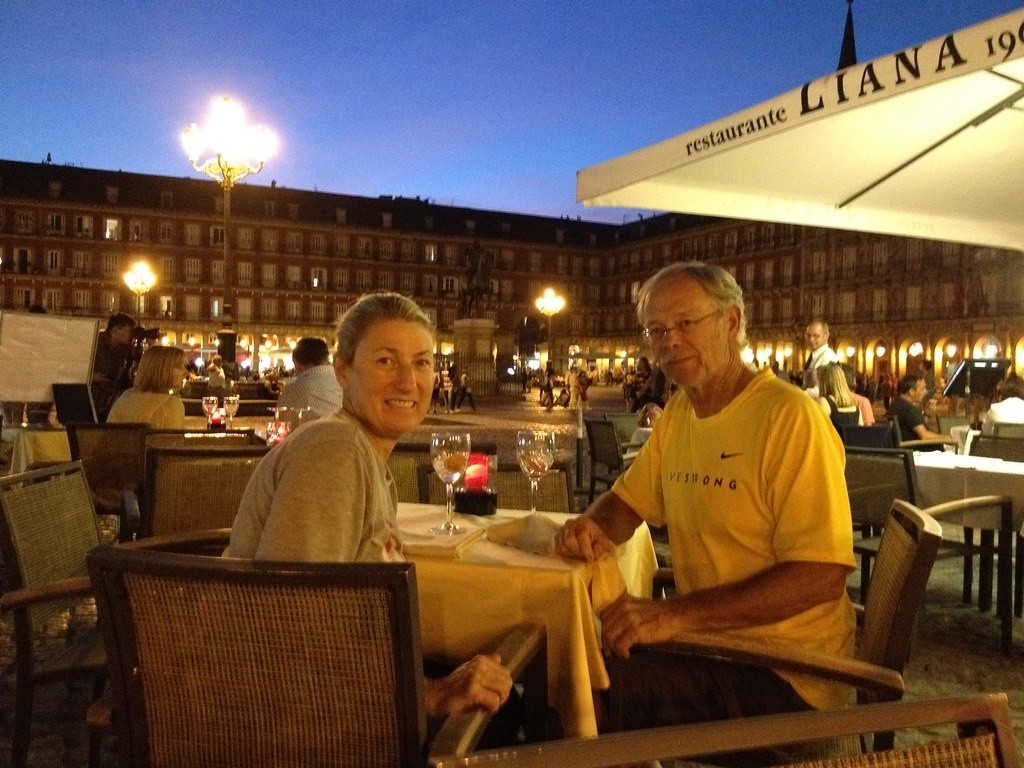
[
  {"left": 396, "top": 500, "right": 647, "bottom": 682},
  {"left": 184, "top": 424, "right": 283, "bottom": 449},
  {"left": 9, "top": 419, "right": 78, "bottom": 474},
  {"left": 900, "top": 448, "right": 1024, "bottom": 605}
]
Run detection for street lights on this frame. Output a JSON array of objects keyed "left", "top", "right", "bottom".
[
  {"left": 181, "top": 93, "right": 281, "bottom": 380},
  {"left": 122, "top": 260, "right": 156, "bottom": 326},
  {"left": 533, "top": 287, "right": 567, "bottom": 360}
]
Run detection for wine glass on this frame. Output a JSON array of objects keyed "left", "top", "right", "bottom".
[
  {"left": 429, "top": 432, "right": 471, "bottom": 537},
  {"left": 515, "top": 432, "right": 557, "bottom": 514},
  {"left": 223, "top": 396, "right": 241, "bottom": 430},
  {"left": 201, "top": 396, "right": 218, "bottom": 430}
]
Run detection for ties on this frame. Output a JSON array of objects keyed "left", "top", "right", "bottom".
[{"left": 803, "top": 351, "right": 813, "bottom": 370}]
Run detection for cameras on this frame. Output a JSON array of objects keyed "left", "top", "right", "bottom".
[{"left": 132, "top": 325, "right": 160, "bottom": 340}]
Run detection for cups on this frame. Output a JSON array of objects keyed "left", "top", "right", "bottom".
[{"left": 265, "top": 421, "right": 291, "bottom": 443}]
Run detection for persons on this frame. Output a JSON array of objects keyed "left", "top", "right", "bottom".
[
  {"left": 228, "top": 291, "right": 515, "bottom": 719},
  {"left": 461, "top": 235, "right": 481, "bottom": 284},
  {"left": 553, "top": 261, "right": 856, "bottom": 768},
  {"left": 2, "top": 306, "right": 1023, "bottom": 451}
]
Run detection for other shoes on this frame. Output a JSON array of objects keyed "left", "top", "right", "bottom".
[{"left": 425, "top": 408, "right": 481, "bottom": 415}]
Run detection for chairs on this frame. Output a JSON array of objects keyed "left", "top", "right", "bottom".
[{"left": 0, "top": 375, "right": 1024, "bottom": 768}]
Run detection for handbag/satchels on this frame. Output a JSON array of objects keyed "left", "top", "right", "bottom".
[{"left": 465, "top": 382, "right": 473, "bottom": 394}]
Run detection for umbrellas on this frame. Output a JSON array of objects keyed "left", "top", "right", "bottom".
[{"left": 575, "top": 7, "right": 1024, "bottom": 252}]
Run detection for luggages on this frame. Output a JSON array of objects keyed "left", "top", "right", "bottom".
[
  {"left": 538, "top": 390, "right": 554, "bottom": 408},
  {"left": 556, "top": 387, "right": 571, "bottom": 409}
]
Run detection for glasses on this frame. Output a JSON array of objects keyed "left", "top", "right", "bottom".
[
  {"left": 642, "top": 308, "right": 719, "bottom": 345},
  {"left": 804, "top": 332, "right": 825, "bottom": 340}
]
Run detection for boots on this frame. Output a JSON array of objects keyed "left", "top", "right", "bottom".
[{"left": 572, "top": 400, "right": 593, "bottom": 412}]
